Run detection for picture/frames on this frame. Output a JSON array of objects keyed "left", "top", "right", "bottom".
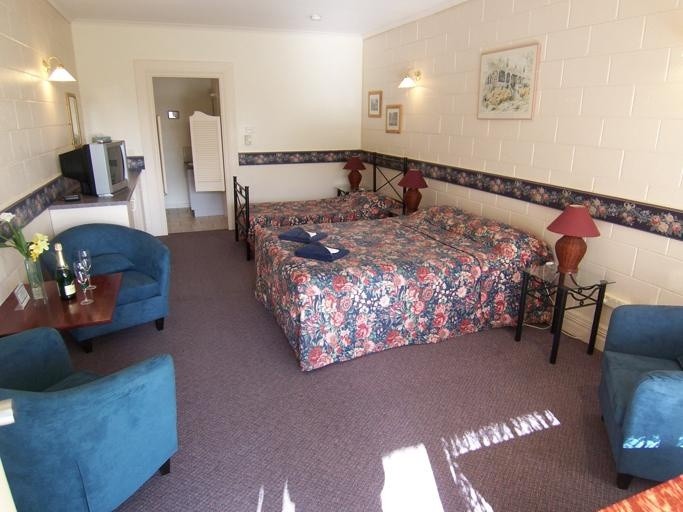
[
  {"left": 64, "top": 91, "right": 82, "bottom": 149},
  {"left": 473, "top": 41, "right": 541, "bottom": 121},
  {"left": 367, "top": 90, "right": 382, "bottom": 117},
  {"left": 167, "top": 110, "right": 178, "bottom": 119},
  {"left": 384, "top": 103, "right": 403, "bottom": 133}
]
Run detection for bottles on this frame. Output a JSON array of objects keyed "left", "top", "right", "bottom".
[
  {"left": 63, "top": 301, "right": 85, "bottom": 325},
  {"left": 51, "top": 243, "right": 76, "bottom": 301}
]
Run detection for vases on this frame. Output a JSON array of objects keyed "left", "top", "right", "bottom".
[{"left": 22, "top": 256, "right": 51, "bottom": 308}]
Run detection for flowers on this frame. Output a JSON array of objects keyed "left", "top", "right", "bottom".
[{"left": 0, "top": 211, "right": 51, "bottom": 264}]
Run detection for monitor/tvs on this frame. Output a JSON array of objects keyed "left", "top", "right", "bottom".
[{"left": 59, "top": 140, "right": 128, "bottom": 197}]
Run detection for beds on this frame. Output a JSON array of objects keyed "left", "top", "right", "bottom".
[
  {"left": 231, "top": 152, "right": 408, "bottom": 262},
  {"left": 250, "top": 204, "right": 554, "bottom": 374}
]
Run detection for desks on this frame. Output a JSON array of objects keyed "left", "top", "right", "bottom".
[
  {"left": 513, "top": 264, "right": 617, "bottom": 365},
  {"left": 335, "top": 185, "right": 372, "bottom": 197},
  {"left": 47, "top": 168, "right": 146, "bottom": 232},
  {"left": 0, "top": 270, "right": 125, "bottom": 339},
  {"left": 384, "top": 206, "right": 424, "bottom": 216}
]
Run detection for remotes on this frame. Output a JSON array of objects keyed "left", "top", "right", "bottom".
[{"left": 97, "top": 140, "right": 112, "bottom": 144}]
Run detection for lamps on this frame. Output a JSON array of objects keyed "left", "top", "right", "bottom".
[
  {"left": 341, "top": 157, "right": 366, "bottom": 190},
  {"left": 396, "top": 70, "right": 421, "bottom": 89},
  {"left": 396, "top": 167, "right": 429, "bottom": 211},
  {"left": 41, "top": 56, "right": 77, "bottom": 82},
  {"left": 545, "top": 204, "right": 601, "bottom": 276}
]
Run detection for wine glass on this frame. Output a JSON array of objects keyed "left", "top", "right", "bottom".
[{"left": 72, "top": 247, "right": 96, "bottom": 305}]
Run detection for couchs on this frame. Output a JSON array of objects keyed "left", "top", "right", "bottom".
[
  {"left": 0, "top": 324, "right": 182, "bottom": 512},
  {"left": 597, "top": 304, "right": 681, "bottom": 491},
  {"left": 37, "top": 223, "right": 172, "bottom": 354}
]
